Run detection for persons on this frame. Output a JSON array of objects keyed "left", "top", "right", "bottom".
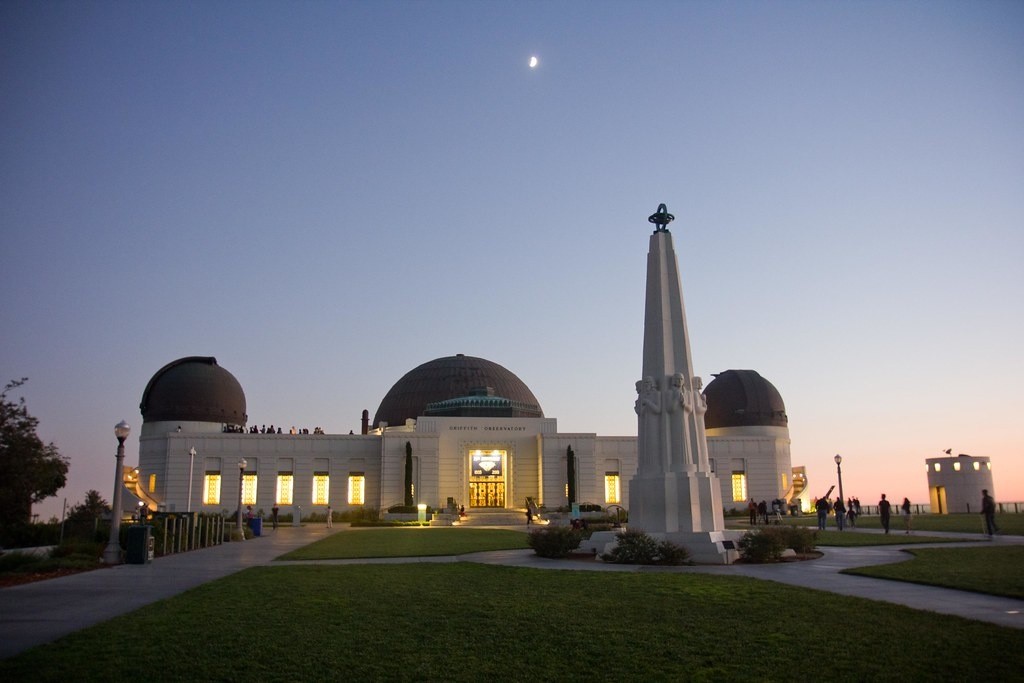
[
  {"left": 748, "top": 497, "right": 781, "bottom": 525},
  {"left": 693, "top": 376, "right": 708, "bottom": 464},
  {"left": 327, "top": 506, "right": 334, "bottom": 528},
  {"left": 290, "top": 426, "right": 309, "bottom": 434},
  {"left": 134, "top": 502, "right": 149, "bottom": 521},
  {"left": 246, "top": 508, "right": 254, "bottom": 528},
  {"left": 833, "top": 497, "right": 846, "bottom": 530},
  {"left": 222, "top": 425, "right": 243, "bottom": 433},
  {"left": 349, "top": 430, "right": 354, "bottom": 434},
  {"left": 260, "top": 425, "right": 282, "bottom": 434},
  {"left": 981, "top": 489, "right": 996, "bottom": 536},
  {"left": 902, "top": 498, "right": 911, "bottom": 534},
  {"left": 878, "top": 494, "right": 891, "bottom": 535},
  {"left": 272, "top": 504, "right": 280, "bottom": 531},
  {"left": 667, "top": 373, "right": 693, "bottom": 464},
  {"left": 814, "top": 496, "right": 832, "bottom": 530},
  {"left": 634, "top": 375, "right": 661, "bottom": 465},
  {"left": 846, "top": 495, "right": 861, "bottom": 531},
  {"left": 458, "top": 504, "right": 468, "bottom": 518},
  {"left": 525, "top": 505, "right": 535, "bottom": 529},
  {"left": 250, "top": 424, "right": 259, "bottom": 434},
  {"left": 313, "top": 426, "right": 324, "bottom": 434}
]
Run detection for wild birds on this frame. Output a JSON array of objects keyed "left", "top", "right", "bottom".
[{"left": 943, "top": 449, "right": 951, "bottom": 456}]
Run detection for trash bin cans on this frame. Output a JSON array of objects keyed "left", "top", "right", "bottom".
[
  {"left": 251, "top": 517, "right": 262, "bottom": 536},
  {"left": 791, "top": 505, "right": 798, "bottom": 516},
  {"left": 127, "top": 525, "right": 155, "bottom": 564}
]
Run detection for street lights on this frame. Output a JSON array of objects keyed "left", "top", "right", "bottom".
[
  {"left": 232, "top": 456, "right": 248, "bottom": 541},
  {"left": 99, "top": 418, "right": 132, "bottom": 566},
  {"left": 835, "top": 453, "right": 845, "bottom": 505},
  {"left": 187, "top": 445, "right": 197, "bottom": 513}
]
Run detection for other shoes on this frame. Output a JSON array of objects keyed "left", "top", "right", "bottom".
[
  {"left": 984, "top": 534, "right": 993, "bottom": 538},
  {"left": 885, "top": 528, "right": 889, "bottom": 534},
  {"left": 840, "top": 528, "right": 842, "bottom": 531}
]
